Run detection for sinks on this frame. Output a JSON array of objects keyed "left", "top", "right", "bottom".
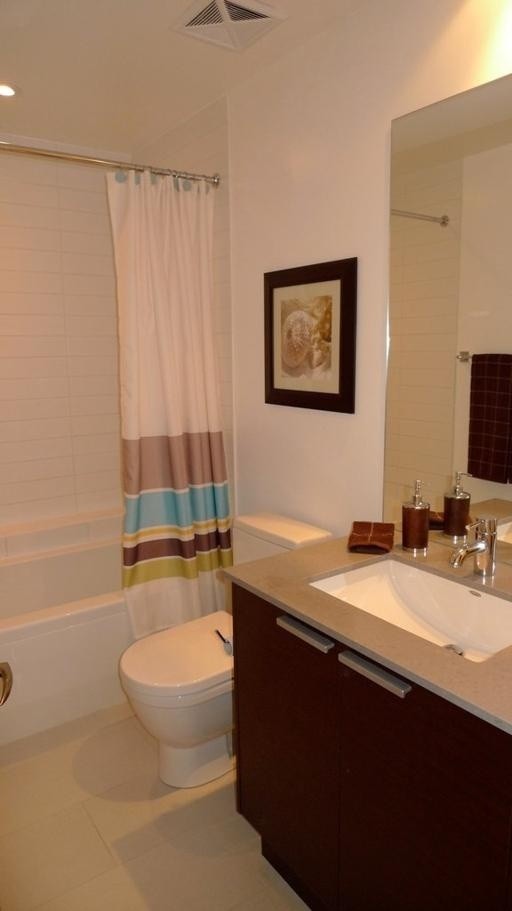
[
  {"left": 305, "top": 551, "right": 512, "bottom": 665},
  {"left": 490, "top": 515, "right": 512, "bottom": 545}
]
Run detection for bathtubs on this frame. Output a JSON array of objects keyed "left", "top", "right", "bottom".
[{"left": 1, "top": 516, "right": 333, "bottom": 744}]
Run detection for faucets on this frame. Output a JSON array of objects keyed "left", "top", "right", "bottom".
[{"left": 448, "top": 515, "right": 500, "bottom": 579}]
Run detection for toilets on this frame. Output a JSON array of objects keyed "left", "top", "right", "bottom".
[{"left": 118, "top": 512, "right": 334, "bottom": 788}]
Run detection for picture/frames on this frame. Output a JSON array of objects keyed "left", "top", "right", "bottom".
[{"left": 261, "top": 253, "right": 359, "bottom": 416}]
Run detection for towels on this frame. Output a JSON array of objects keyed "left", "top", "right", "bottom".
[
  {"left": 345, "top": 519, "right": 396, "bottom": 556},
  {"left": 427, "top": 507, "right": 476, "bottom": 531},
  {"left": 463, "top": 351, "right": 512, "bottom": 486}
]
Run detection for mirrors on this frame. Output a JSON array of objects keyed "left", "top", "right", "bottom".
[{"left": 376, "top": 71, "right": 512, "bottom": 603}]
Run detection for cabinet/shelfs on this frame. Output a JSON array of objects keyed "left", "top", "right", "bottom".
[{"left": 227, "top": 577, "right": 512, "bottom": 910}]
